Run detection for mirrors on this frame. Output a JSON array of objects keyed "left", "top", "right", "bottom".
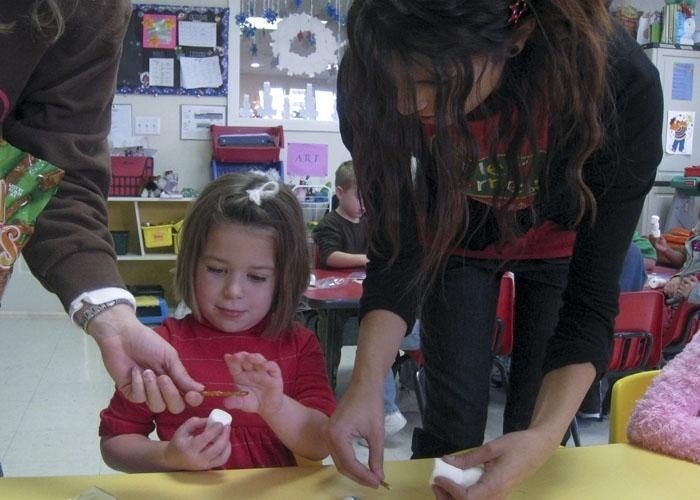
[{"left": 226, "top": 0, "right": 353, "bottom": 133}]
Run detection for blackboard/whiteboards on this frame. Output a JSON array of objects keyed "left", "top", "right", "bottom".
[{"left": 115, "top": 4, "right": 230, "bottom": 96}]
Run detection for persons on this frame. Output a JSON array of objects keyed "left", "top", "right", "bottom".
[
  {"left": 312, "top": 161, "right": 369, "bottom": 383},
  {"left": 99, "top": 171, "right": 336, "bottom": 473},
  {"left": 0, "top": 0, "right": 205, "bottom": 415},
  {"left": 357, "top": 320, "right": 420, "bottom": 445},
  {"left": 577, "top": 227, "right": 700, "bottom": 465},
  {"left": 322, "top": 0, "right": 662, "bottom": 499}
]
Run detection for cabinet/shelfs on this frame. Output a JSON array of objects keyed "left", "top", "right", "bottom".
[{"left": 104, "top": 193, "right": 202, "bottom": 308}]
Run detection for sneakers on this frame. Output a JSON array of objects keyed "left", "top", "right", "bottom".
[
  {"left": 491, "top": 354, "right": 509, "bottom": 388},
  {"left": 577, "top": 394, "right": 601, "bottom": 419},
  {"left": 357, "top": 409, "right": 407, "bottom": 447}
]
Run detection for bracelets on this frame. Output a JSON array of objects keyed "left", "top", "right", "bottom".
[{"left": 80, "top": 299, "right": 133, "bottom": 333}]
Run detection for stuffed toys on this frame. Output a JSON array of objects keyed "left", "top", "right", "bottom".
[{"left": 137, "top": 170, "right": 183, "bottom": 198}]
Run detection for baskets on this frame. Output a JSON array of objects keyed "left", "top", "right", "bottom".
[{"left": 109, "top": 157, "right": 153, "bottom": 196}]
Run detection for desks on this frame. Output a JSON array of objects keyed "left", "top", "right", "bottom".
[{"left": 1, "top": 442, "right": 700, "bottom": 500}]
